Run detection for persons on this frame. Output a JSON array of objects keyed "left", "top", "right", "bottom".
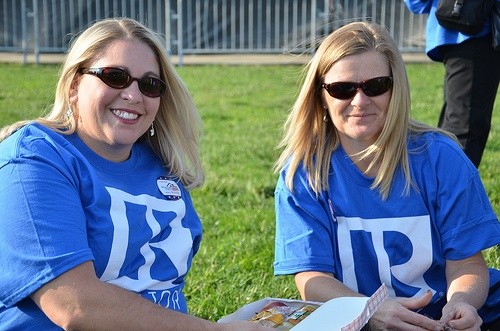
[
  {"left": 270, "top": 21, "right": 500, "bottom": 331},
  {"left": 0, "top": 18, "right": 277, "bottom": 331},
  {"left": 404, "top": 0, "right": 500, "bottom": 169}
]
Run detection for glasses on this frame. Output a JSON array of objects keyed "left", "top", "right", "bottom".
[
  {"left": 321, "top": 76, "right": 393, "bottom": 100},
  {"left": 77, "top": 67, "right": 167, "bottom": 98}
]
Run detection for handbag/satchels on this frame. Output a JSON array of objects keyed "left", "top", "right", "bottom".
[{"left": 435, "top": 0, "right": 492, "bottom": 35}]
